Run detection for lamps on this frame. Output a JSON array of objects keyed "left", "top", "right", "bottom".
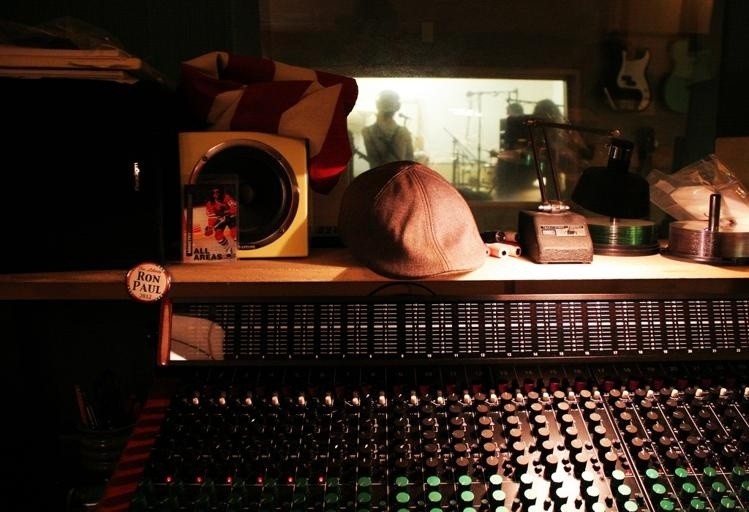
[{"left": 518, "top": 115, "right": 650, "bottom": 266}]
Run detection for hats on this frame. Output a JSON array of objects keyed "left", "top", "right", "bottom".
[{"left": 337, "top": 161, "right": 486, "bottom": 280}]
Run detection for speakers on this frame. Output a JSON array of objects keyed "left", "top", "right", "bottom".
[{"left": 175, "top": 132, "right": 311, "bottom": 260}]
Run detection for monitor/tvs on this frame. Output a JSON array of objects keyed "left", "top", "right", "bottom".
[{"left": 315, "top": 66, "right": 584, "bottom": 234}]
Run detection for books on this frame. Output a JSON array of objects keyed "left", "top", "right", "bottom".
[{"left": 0, "top": 46, "right": 142, "bottom": 79}]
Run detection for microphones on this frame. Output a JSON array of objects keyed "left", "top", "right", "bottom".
[
  {"left": 464, "top": 90, "right": 477, "bottom": 97},
  {"left": 394, "top": 110, "right": 411, "bottom": 122}
]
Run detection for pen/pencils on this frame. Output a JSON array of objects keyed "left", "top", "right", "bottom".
[{"left": 479, "top": 231, "right": 524, "bottom": 259}]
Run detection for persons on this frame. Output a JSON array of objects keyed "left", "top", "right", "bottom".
[
  {"left": 506, "top": 104, "right": 524, "bottom": 116},
  {"left": 362, "top": 90, "right": 414, "bottom": 168},
  {"left": 533, "top": 99, "right": 562, "bottom": 115},
  {"left": 206, "top": 186, "right": 237, "bottom": 257}
]
